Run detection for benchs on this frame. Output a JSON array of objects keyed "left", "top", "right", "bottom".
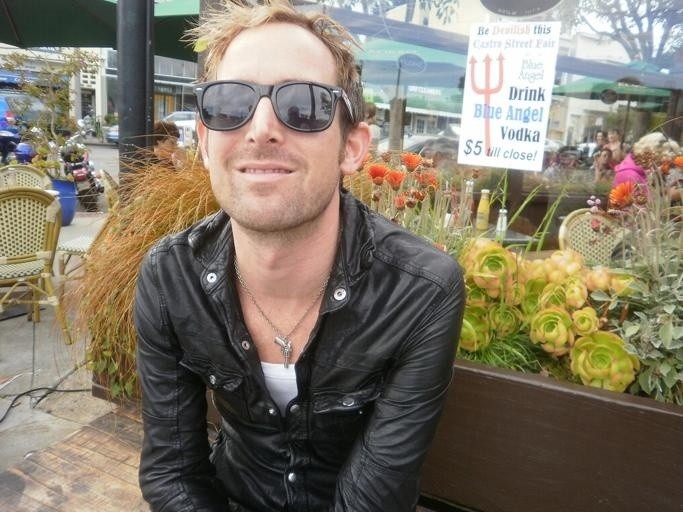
[{"left": 414, "top": 357, "right": 682, "bottom": 512}]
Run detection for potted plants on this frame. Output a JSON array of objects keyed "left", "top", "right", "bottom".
[{"left": 0, "top": 46, "right": 104, "bottom": 226}]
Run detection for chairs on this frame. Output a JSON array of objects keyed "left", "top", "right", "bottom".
[
  {"left": 558, "top": 208, "right": 627, "bottom": 266},
  {"left": 0, "top": 188, "right": 72, "bottom": 344},
  {"left": 58, "top": 181, "right": 123, "bottom": 317},
  {"left": 0, "top": 164, "right": 72, "bottom": 345}
]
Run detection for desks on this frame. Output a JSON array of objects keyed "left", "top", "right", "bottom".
[{"left": 442, "top": 212, "right": 542, "bottom": 246}]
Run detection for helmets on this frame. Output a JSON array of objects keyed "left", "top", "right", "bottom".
[
  {"left": 15, "top": 142, "right": 33, "bottom": 161},
  {"left": 59, "top": 148, "right": 84, "bottom": 162}
]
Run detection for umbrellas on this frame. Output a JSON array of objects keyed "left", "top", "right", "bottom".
[{"left": 551, "top": 59, "right": 671, "bottom": 162}]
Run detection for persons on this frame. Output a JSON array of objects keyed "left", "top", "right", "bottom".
[
  {"left": 282, "top": 105, "right": 311, "bottom": 131},
  {"left": 590, "top": 130, "right": 608, "bottom": 159},
  {"left": 609, "top": 132, "right": 670, "bottom": 221},
  {"left": 132, "top": 3, "right": 467, "bottom": 511},
  {"left": 150, "top": 116, "right": 180, "bottom": 174},
  {"left": 588, "top": 148, "right": 614, "bottom": 184},
  {"left": 602, "top": 127, "right": 632, "bottom": 166},
  {"left": 664, "top": 164, "right": 682, "bottom": 222}
]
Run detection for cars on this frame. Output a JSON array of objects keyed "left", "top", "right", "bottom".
[
  {"left": 378, "top": 133, "right": 459, "bottom": 161},
  {"left": 0, "top": 88, "right": 55, "bottom": 162},
  {"left": 163, "top": 111, "right": 197, "bottom": 136}
]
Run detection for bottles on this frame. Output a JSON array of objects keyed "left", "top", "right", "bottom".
[{"left": 464, "top": 181, "right": 508, "bottom": 232}]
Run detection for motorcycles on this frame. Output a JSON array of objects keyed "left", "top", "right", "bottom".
[{"left": 59, "top": 119, "right": 104, "bottom": 212}]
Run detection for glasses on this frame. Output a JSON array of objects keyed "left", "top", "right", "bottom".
[{"left": 191, "top": 78, "right": 355, "bottom": 133}]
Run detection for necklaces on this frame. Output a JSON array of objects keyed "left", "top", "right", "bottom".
[{"left": 229, "top": 254, "right": 334, "bottom": 370}]
